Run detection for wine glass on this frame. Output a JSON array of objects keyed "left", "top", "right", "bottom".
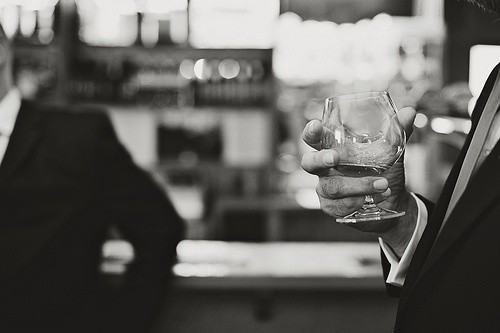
[{"left": 322, "top": 89, "right": 410, "bottom": 225}]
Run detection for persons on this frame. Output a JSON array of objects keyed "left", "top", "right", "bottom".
[
  {"left": 299, "top": 0, "right": 499, "bottom": 333},
  {"left": 0, "top": 22, "right": 185, "bottom": 333}
]
